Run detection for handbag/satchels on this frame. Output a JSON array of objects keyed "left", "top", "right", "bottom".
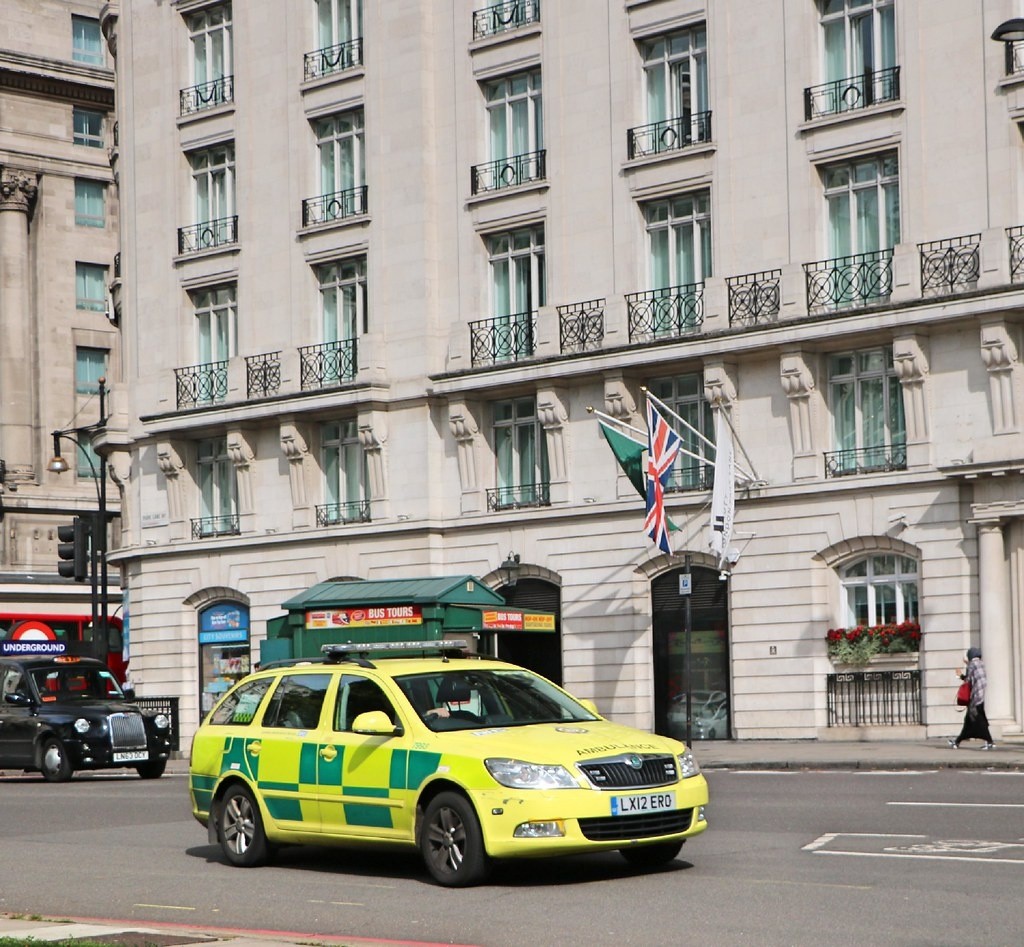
[{"left": 953, "top": 678, "right": 971, "bottom": 712}]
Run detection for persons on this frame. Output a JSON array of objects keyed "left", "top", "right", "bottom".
[
  {"left": 419, "top": 707, "right": 450, "bottom": 720},
  {"left": 254, "top": 661, "right": 261, "bottom": 672},
  {"left": 946, "top": 647, "right": 997, "bottom": 750}
]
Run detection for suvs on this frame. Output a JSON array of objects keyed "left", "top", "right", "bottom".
[{"left": 0, "top": 655, "right": 171, "bottom": 781}]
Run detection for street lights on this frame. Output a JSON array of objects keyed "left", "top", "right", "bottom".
[{"left": 48, "top": 377, "right": 109, "bottom": 695}]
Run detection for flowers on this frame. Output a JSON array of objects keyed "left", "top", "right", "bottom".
[{"left": 825, "top": 620, "right": 922, "bottom": 665}]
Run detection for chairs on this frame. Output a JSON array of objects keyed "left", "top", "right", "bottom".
[{"left": 437, "top": 677, "right": 482, "bottom": 721}]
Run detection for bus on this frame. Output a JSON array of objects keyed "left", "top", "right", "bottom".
[{"left": 0, "top": 613, "right": 135, "bottom": 700}]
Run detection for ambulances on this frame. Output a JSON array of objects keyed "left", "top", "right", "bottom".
[{"left": 189, "top": 640, "right": 708, "bottom": 883}]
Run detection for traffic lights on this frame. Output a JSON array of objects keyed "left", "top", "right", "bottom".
[{"left": 58, "top": 516, "right": 86, "bottom": 582}]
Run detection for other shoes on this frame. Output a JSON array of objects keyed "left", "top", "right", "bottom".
[
  {"left": 980, "top": 742, "right": 997, "bottom": 750},
  {"left": 948, "top": 739, "right": 959, "bottom": 749}
]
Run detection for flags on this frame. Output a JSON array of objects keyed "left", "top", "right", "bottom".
[
  {"left": 706, "top": 407, "right": 735, "bottom": 571},
  {"left": 596, "top": 419, "right": 680, "bottom": 532},
  {"left": 642, "top": 398, "right": 685, "bottom": 556}
]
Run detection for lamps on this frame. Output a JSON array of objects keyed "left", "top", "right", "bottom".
[{"left": 499, "top": 551, "right": 521, "bottom": 571}]
